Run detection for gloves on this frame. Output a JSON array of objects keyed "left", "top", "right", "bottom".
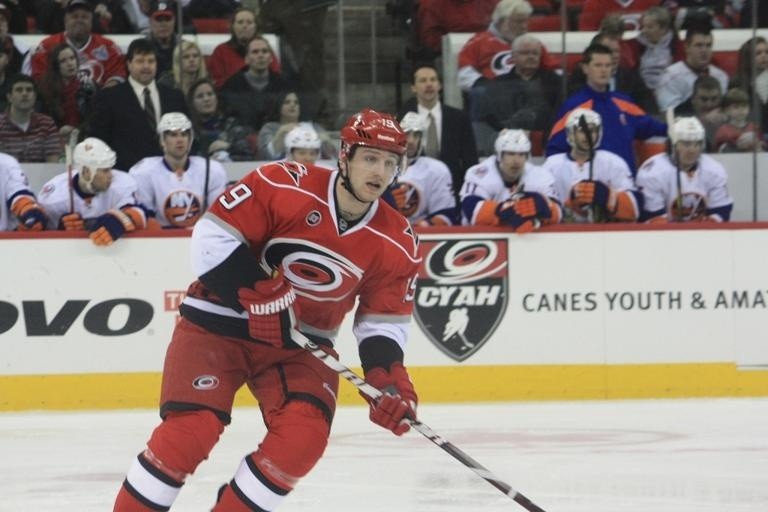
[
  {"left": 60, "top": 212, "right": 81, "bottom": 230},
  {"left": 413, "top": 215, "right": 449, "bottom": 231},
  {"left": 495, "top": 191, "right": 557, "bottom": 230},
  {"left": 364, "top": 361, "right": 419, "bottom": 438},
  {"left": 570, "top": 179, "right": 618, "bottom": 213},
  {"left": 385, "top": 183, "right": 409, "bottom": 211},
  {"left": 238, "top": 265, "right": 302, "bottom": 351},
  {"left": 90, "top": 214, "right": 126, "bottom": 244},
  {"left": 10, "top": 197, "right": 45, "bottom": 232}
]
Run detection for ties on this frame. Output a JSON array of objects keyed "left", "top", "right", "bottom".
[
  {"left": 142, "top": 87, "right": 156, "bottom": 135},
  {"left": 423, "top": 113, "right": 441, "bottom": 158}
]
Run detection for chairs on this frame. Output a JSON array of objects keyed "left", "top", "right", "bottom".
[{"left": 0, "top": 2, "right": 768, "bottom": 230}]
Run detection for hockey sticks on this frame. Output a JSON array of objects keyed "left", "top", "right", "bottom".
[
  {"left": 582, "top": 113, "right": 596, "bottom": 225},
  {"left": 194, "top": 138, "right": 210, "bottom": 212},
  {"left": 664, "top": 107, "right": 683, "bottom": 220},
  {"left": 64, "top": 145, "right": 75, "bottom": 213}
]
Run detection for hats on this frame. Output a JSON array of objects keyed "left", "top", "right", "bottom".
[
  {"left": 148, "top": 0, "right": 176, "bottom": 17},
  {"left": 63, "top": 0, "right": 95, "bottom": 14}
]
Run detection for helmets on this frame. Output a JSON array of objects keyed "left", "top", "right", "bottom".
[
  {"left": 668, "top": 117, "right": 706, "bottom": 144},
  {"left": 155, "top": 111, "right": 192, "bottom": 134},
  {"left": 494, "top": 127, "right": 531, "bottom": 163},
  {"left": 398, "top": 110, "right": 428, "bottom": 133},
  {"left": 340, "top": 105, "right": 408, "bottom": 155},
  {"left": 71, "top": 137, "right": 118, "bottom": 170},
  {"left": 567, "top": 107, "right": 601, "bottom": 131},
  {"left": 283, "top": 121, "right": 323, "bottom": 150}
]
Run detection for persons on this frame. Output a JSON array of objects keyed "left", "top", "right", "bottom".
[{"left": 112, "top": 108, "right": 424, "bottom": 512}]
